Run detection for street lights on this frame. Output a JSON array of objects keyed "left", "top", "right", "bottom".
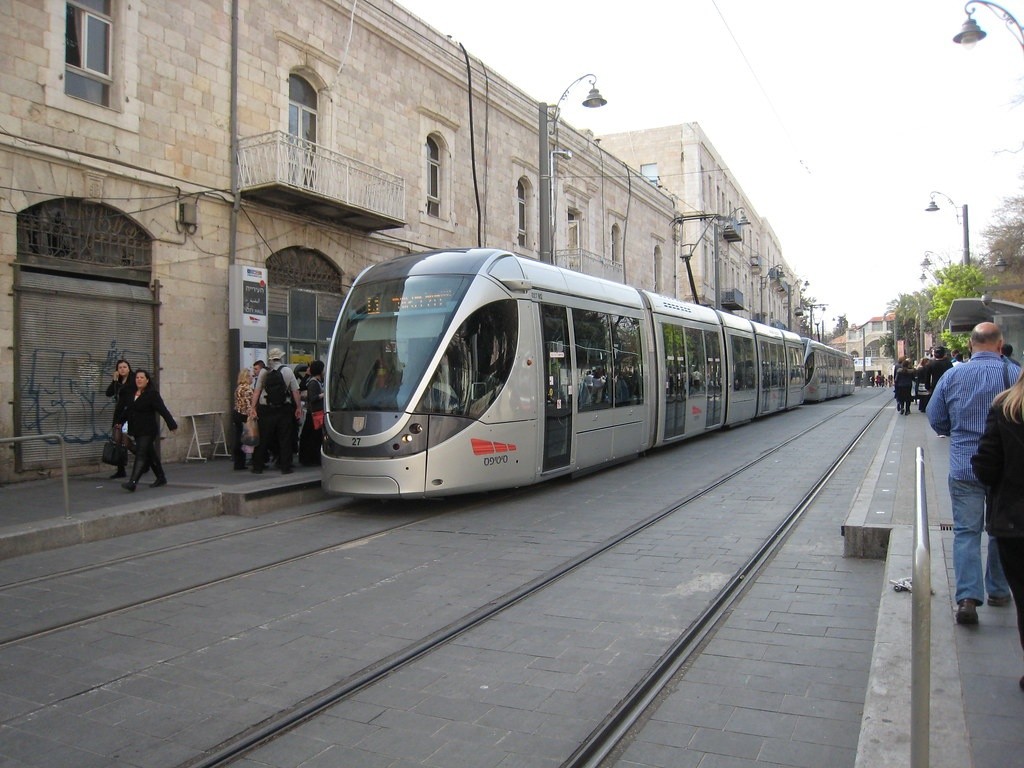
[
  {"left": 714, "top": 208, "right": 751, "bottom": 311},
  {"left": 788, "top": 275, "right": 810, "bottom": 332},
  {"left": 926, "top": 191, "right": 970, "bottom": 267},
  {"left": 810, "top": 305, "right": 826, "bottom": 340},
  {"left": 759, "top": 264, "right": 787, "bottom": 324},
  {"left": 540, "top": 74, "right": 607, "bottom": 264}
]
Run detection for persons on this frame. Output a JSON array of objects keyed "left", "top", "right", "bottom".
[
  {"left": 867, "top": 320, "right": 1021, "bottom": 438},
  {"left": 584, "top": 365, "right": 704, "bottom": 400},
  {"left": 104, "top": 360, "right": 149, "bottom": 479},
  {"left": 971, "top": 355, "right": 1024, "bottom": 685},
  {"left": 923, "top": 320, "right": 1023, "bottom": 625},
  {"left": 114, "top": 369, "right": 179, "bottom": 491},
  {"left": 232, "top": 348, "right": 324, "bottom": 475}
]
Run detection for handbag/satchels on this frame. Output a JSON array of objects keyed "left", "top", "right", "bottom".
[
  {"left": 311, "top": 410, "right": 324, "bottom": 430},
  {"left": 240, "top": 415, "right": 261, "bottom": 447},
  {"left": 917, "top": 383, "right": 930, "bottom": 395},
  {"left": 102, "top": 442, "right": 129, "bottom": 467}
]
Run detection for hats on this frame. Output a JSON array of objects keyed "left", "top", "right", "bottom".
[
  {"left": 934, "top": 347, "right": 946, "bottom": 353},
  {"left": 267, "top": 348, "right": 286, "bottom": 360}
]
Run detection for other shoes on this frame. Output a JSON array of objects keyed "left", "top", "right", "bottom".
[
  {"left": 282, "top": 470, "right": 293, "bottom": 474},
  {"left": 110, "top": 467, "right": 126, "bottom": 479},
  {"left": 987, "top": 592, "right": 1011, "bottom": 606},
  {"left": 121, "top": 481, "right": 136, "bottom": 492},
  {"left": 251, "top": 469, "right": 262, "bottom": 474},
  {"left": 149, "top": 477, "right": 167, "bottom": 487},
  {"left": 937, "top": 433, "right": 946, "bottom": 438},
  {"left": 956, "top": 599, "right": 978, "bottom": 625},
  {"left": 233, "top": 467, "right": 248, "bottom": 470}
]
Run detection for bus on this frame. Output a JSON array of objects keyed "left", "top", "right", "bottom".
[
  {"left": 321, "top": 249, "right": 855, "bottom": 500},
  {"left": 736, "top": 360, "right": 785, "bottom": 388}
]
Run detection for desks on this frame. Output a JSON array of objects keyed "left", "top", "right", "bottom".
[{"left": 182, "top": 411, "right": 232, "bottom": 463}]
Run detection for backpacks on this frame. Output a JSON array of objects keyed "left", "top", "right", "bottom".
[{"left": 262, "top": 365, "right": 287, "bottom": 407}]
